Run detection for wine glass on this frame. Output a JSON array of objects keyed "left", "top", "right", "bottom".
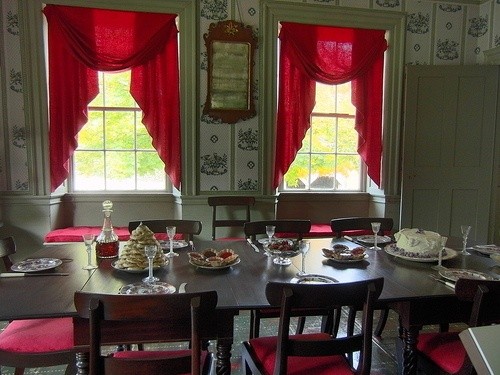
[
  {"left": 81, "top": 233, "right": 99, "bottom": 269},
  {"left": 164, "top": 226, "right": 179, "bottom": 258},
  {"left": 458, "top": 225, "right": 472, "bottom": 255},
  {"left": 296, "top": 241, "right": 311, "bottom": 276},
  {"left": 142, "top": 244, "right": 160, "bottom": 282},
  {"left": 266, "top": 226, "right": 275, "bottom": 243},
  {"left": 370, "top": 222, "right": 382, "bottom": 250},
  {"left": 431, "top": 236, "right": 448, "bottom": 271}
]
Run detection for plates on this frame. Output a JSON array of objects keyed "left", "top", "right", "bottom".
[
  {"left": 119, "top": 282, "right": 176, "bottom": 295},
  {"left": 11, "top": 258, "right": 63, "bottom": 272},
  {"left": 159, "top": 241, "right": 188, "bottom": 248},
  {"left": 188, "top": 256, "right": 241, "bottom": 269},
  {"left": 111, "top": 258, "right": 166, "bottom": 271},
  {"left": 260, "top": 237, "right": 500, "bottom": 284}
]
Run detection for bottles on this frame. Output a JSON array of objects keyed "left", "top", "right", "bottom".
[{"left": 96, "top": 200, "right": 120, "bottom": 258}]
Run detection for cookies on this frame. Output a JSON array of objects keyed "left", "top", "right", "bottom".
[{"left": 113, "top": 222, "right": 165, "bottom": 270}]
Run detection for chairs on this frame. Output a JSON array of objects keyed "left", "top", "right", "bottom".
[{"left": 0, "top": 195, "right": 490, "bottom": 375}]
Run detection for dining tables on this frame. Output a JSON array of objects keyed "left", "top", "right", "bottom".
[{"left": 0, "top": 236, "right": 500, "bottom": 375}]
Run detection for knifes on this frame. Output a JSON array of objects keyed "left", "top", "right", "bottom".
[
  {"left": 189, "top": 240, "right": 196, "bottom": 252},
  {"left": 247, "top": 238, "right": 260, "bottom": 253},
  {"left": 343, "top": 234, "right": 367, "bottom": 248},
  {"left": 0, "top": 272, "right": 70, "bottom": 276},
  {"left": 428, "top": 273, "right": 455, "bottom": 289},
  {"left": 179, "top": 282, "right": 188, "bottom": 294}
]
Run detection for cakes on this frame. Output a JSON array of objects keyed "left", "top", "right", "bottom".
[{"left": 393, "top": 228, "right": 448, "bottom": 258}]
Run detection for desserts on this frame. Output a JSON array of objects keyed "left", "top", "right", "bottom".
[
  {"left": 188, "top": 247, "right": 239, "bottom": 267},
  {"left": 322, "top": 244, "right": 366, "bottom": 259}
]
